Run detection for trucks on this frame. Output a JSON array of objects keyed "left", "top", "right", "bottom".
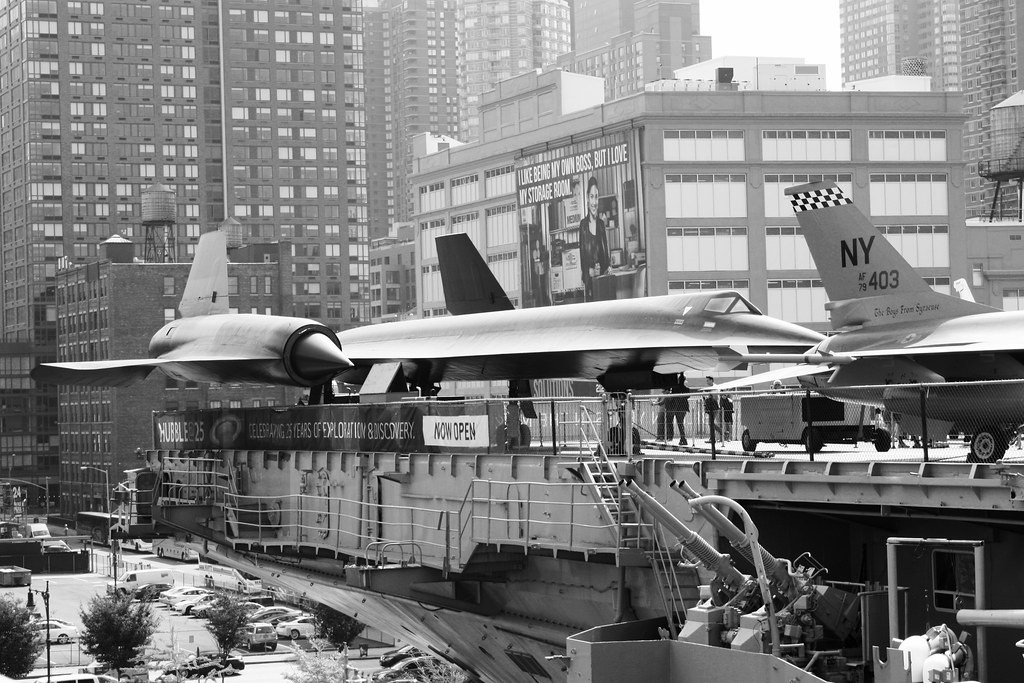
[
  {"left": 106, "top": 569, "right": 175, "bottom": 599},
  {"left": 26, "top": 523, "right": 51, "bottom": 538},
  {"left": 740, "top": 392, "right": 891, "bottom": 454}
]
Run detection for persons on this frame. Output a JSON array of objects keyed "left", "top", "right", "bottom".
[
  {"left": 649, "top": 389, "right": 671, "bottom": 443},
  {"left": 767, "top": 380, "right": 787, "bottom": 394},
  {"left": 697, "top": 375, "right": 730, "bottom": 443},
  {"left": 666, "top": 372, "right": 694, "bottom": 445},
  {"left": 502, "top": 399, "right": 522, "bottom": 453},
  {"left": 605, "top": 393, "right": 642, "bottom": 456},
  {"left": 579, "top": 176, "right": 613, "bottom": 303},
  {"left": 721, "top": 391, "right": 734, "bottom": 442},
  {"left": 532, "top": 239, "right": 546, "bottom": 296}
]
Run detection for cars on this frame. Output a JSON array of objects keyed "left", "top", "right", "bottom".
[
  {"left": 131, "top": 583, "right": 173, "bottom": 602},
  {"left": 190, "top": 598, "right": 219, "bottom": 618},
  {"left": 235, "top": 623, "right": 278, "bottom": 651},
  {"left": 206, "top": 602, "right": 315, "bottom": 628},
  {"left": 372, "top": 656, "right": 450, "bottom": 683},
  {"left": 34, "top": 674, "right": 119, "bottom": 683},
  {"left": 164, "top": 651, "right": 245, "bottom": 679},
  {"left": 175, "top": 594, "right": 215, "bottom": 616},
  {"left": 379, "top": 644, "right": 441, "bottom": 669},
  {"left": 165, "top": 588, "right": 213, "bottom": 607},
  {"left": 13, "top": 618, "right": 79, "bottom": 644},
  {"left": 159, "top": 587, "right": 196, "bottom": 607},
  {"left": 276, "top": 616, "right": 330, "bottom": 640}
]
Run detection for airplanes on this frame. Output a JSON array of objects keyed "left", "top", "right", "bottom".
[
  {"left": 697, "top": 179, "right": 1024, "bottom": 462},
  {"left": 30, "top": 230, "right": 826, "bottom": 455}
]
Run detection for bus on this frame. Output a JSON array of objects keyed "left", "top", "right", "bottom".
[
  {"left": 152, "top": 537, "right": 199, "bottom": 562},
  {"left": 77, "top": 511, "right": 153, "bottom": 553}
]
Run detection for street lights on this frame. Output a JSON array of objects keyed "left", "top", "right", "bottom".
[
  {"left": 46, "top": 477, "right": 51, "bottom": 524},
  {"left": 81, "top": 467, "right": 109, "bottom": 513},
  {"left": 26, "top": 580, "right": 51, "bottom": 683},
  {"left": 12, "top": 454, "right": 15, "bottom": 467}
]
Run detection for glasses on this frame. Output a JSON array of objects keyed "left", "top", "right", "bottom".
[{"left": 775, "top": 384, "right": 780, "bottom": 386}]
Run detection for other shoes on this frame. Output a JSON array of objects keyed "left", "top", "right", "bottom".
[
  {"left": 705, "top": 439, "right": 712, "bottom": 443},
  {"left": 679, "top": 439, "right": 687, "bottom": 445},
  {"left": 718, "top": 435, "right": 722, "bottom": 441}
]
